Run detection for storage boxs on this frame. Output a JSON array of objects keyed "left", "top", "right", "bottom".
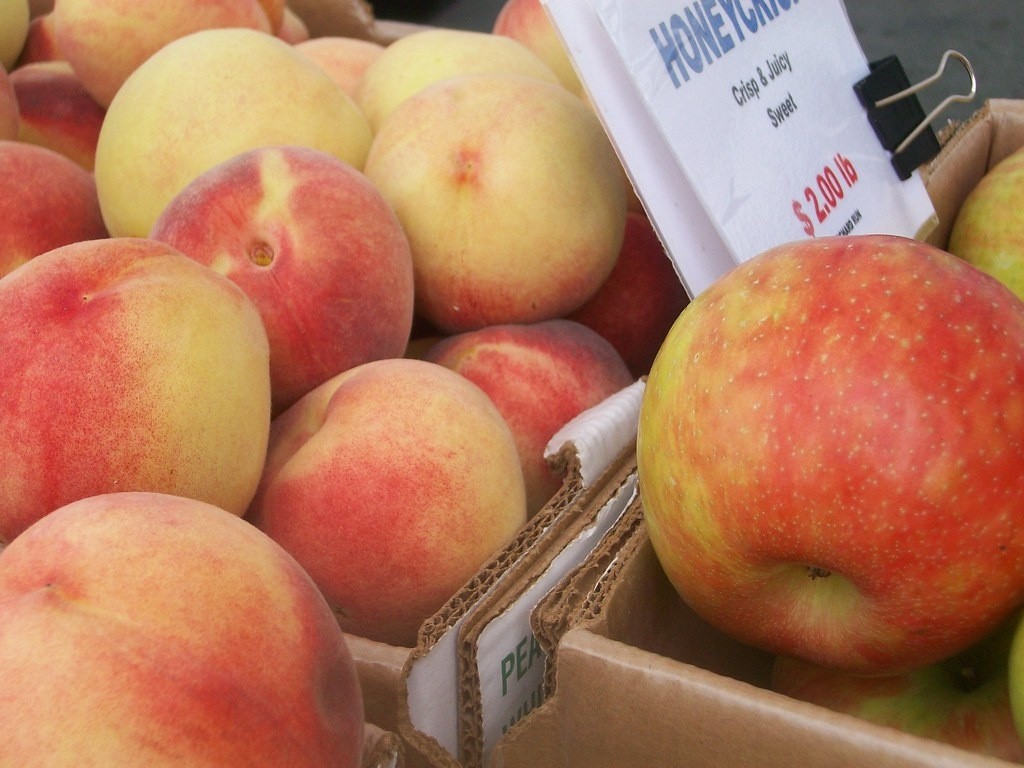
[{"left": 335, "top": 96, "right": 1024, "bottom": 768}]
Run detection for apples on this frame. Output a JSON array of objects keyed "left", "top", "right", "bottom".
[{"left": 637, "top": 145, "right": 1024, "bottom": 764}]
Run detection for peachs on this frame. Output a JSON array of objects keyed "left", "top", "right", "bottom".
[{"left": 0, "top": 0, "right": 681, "bottom": 767}]
